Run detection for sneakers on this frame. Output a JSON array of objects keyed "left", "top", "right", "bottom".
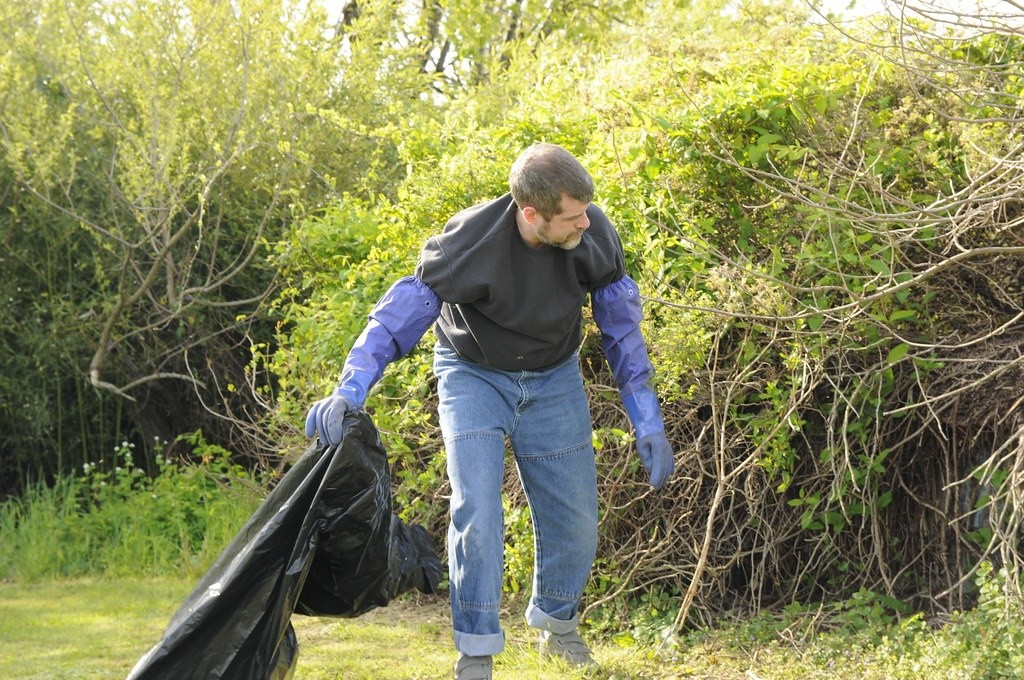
[
  {"left": 454, "top": 651, "right": 493, "bottom": 680},
  {"left": 538, "top": 629, "right": 602, "bottom": 674}
]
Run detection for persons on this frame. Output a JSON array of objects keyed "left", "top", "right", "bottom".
[{"left": 305, "top": 142, "right": 675, "bottom": 680}]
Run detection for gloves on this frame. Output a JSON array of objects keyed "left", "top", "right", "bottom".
[
  {"left": 636, "top": 432, "right": 676, "bottom": 490},
  {"left": 305, "top": 393, "right": 354, "bottom": 449}
]
